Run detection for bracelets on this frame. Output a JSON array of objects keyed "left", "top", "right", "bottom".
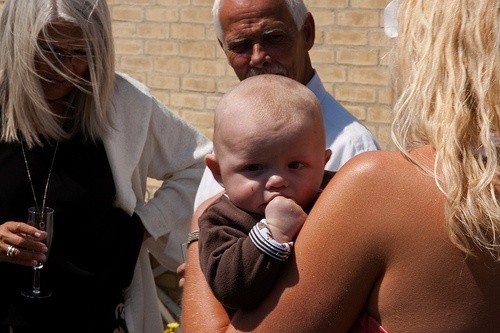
[{"left": 186, "top": 230, "right": 199, "bottom": 249}]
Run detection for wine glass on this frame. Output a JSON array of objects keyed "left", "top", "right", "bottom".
[{"left": 20, "top": 206, "right": 54, "bottom": 300}]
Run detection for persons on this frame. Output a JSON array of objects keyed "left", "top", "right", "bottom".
[
  {"left": 181, "top": 0, "right": 500, "bottom": 333},
  {"left": 192, "top": 0, "right": 380, "bottom": 212},
  {"left": 0, "top": 0, "right": 213, "bottom": 333},
  {"left": 197, "top": 74, "right": 337, "bottom": 312}
]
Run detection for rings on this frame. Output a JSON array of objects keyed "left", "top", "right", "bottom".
[{"left": 7, "top": 245, "right": 15, "bottom": 256}]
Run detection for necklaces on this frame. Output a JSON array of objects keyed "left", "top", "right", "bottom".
[{"left": 19, "top": 133, "right": 59, "bottom": 229}]
